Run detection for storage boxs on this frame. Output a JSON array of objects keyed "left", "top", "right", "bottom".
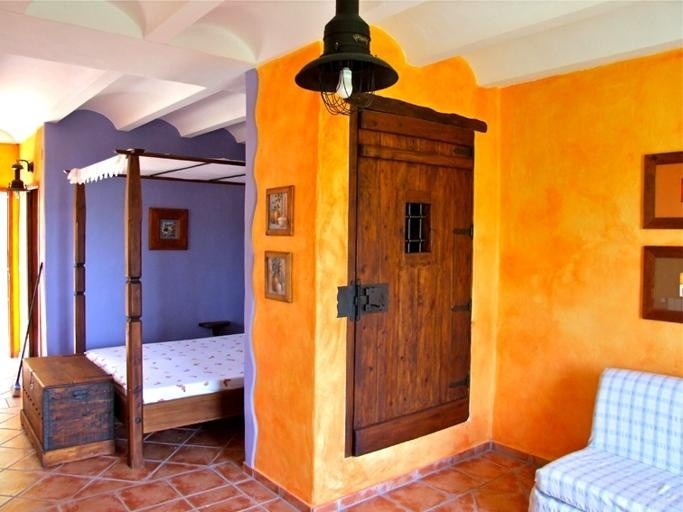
[{"left": 19, "top": 354, "right": 115, "bottom": 469}]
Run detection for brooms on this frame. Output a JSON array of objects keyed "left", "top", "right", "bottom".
[{"left": 9, "top": 262, "right": 44, "bottom": 396}]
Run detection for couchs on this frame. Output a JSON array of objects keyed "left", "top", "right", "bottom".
[{"left": 526, "top": 367, "right": 683, "bottom": 511}]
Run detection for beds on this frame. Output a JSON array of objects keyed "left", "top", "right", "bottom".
[{"left": 83, "top": 334, "right": 243, "bottom": 468}]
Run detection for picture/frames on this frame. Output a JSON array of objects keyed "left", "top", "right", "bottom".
[
  {"left": 148, "top": 208, "right": 189, "bottom": 251},
  {"left": 264, "top": 186, "right": 295, "bottom": 304},
  {"left": 641, "top": 151, "right": 683, "bottom": 327}
]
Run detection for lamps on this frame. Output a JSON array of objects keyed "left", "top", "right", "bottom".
[
  {"left": 286, "top": 2, "right": 399, "bottom": 118},
  {"left": 8, "top": 159, "right": 34, "bottom": 198}
]
